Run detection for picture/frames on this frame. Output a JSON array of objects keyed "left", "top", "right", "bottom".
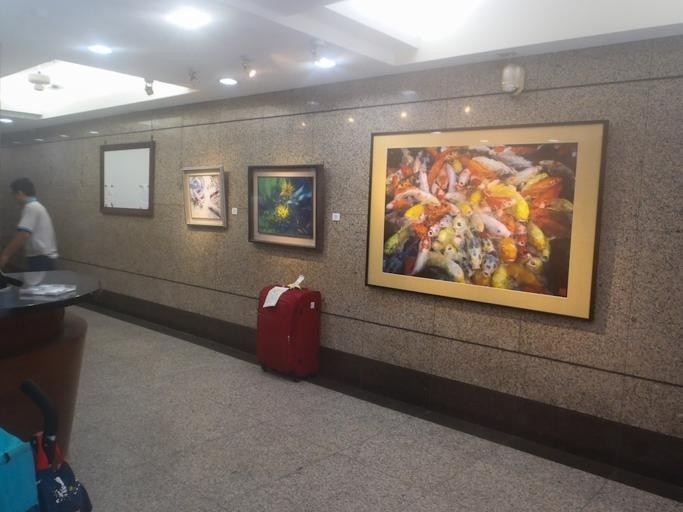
[
  {"left": 100, "top": 140, "right": 155, "bottom": 216},
  {"left": 364, "top": 118, "right": 608, "bottom": 321},
  {"left": 247, "top": 164, "right": 318, "bottom": 250},
  {"left": 182, "top": 164, "right": 227, "bottom": 228}
]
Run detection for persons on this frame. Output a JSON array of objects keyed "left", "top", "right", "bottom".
[{"left": 0, "top": 177, "right": 60, "bottom": 271}]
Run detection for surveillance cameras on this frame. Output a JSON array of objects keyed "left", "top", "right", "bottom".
[{"left": 28, "top": 72, "right": 51, "bottom": 85}]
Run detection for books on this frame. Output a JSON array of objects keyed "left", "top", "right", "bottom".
[{"left": 19, "top": 283, "right": 77, "bottom": 296}]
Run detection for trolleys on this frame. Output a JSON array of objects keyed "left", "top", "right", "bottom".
[{"left": 0, "top": 377, "right": 61, "bottom": 512}]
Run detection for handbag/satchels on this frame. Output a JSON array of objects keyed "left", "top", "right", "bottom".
[{"left": 39, "top": 464, "right": 92, "bottom": 512}]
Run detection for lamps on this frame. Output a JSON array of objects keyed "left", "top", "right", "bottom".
[
  {"left": 144, "top": 71, "right": 154, "bottom": 95},
  {"left": 501, "top": 52, "right": 526, "bottom": 95},
  {"left": 29, "top": 71, "right": 50, "bottom": 90}
]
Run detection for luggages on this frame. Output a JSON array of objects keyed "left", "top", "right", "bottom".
[{"left": 257, "top": 285, "right": 322, "bottom": 382}]
